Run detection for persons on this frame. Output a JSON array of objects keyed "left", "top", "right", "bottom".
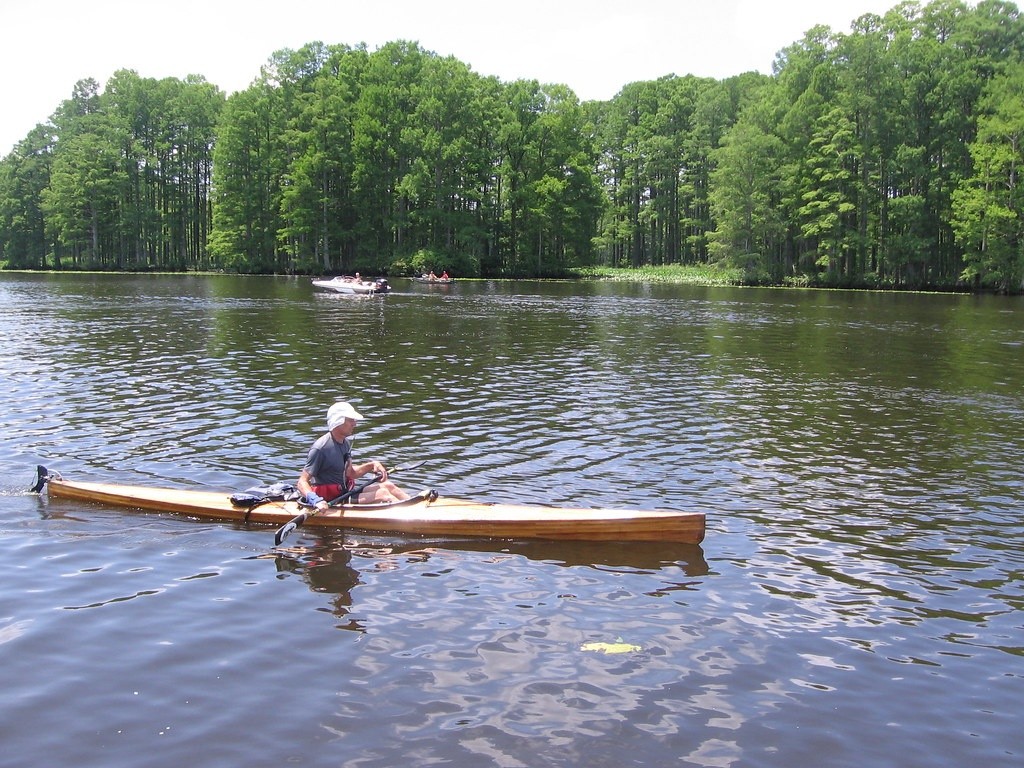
[
  {"left": 297, "top": 400, "right": 410, "bottom": 515},
  {"left": 355, "top": 273, "right": 363, "bottom": 286},
  {"left": 441, "top": 271, "right": 448, "bottom": 280},
  {"left": 430, "top": 270, "right": 438, "bottom": 281}
]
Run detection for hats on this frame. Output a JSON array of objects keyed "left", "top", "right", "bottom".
[{"left": 326, "top": 401, "right": 363, "bottom": 432}]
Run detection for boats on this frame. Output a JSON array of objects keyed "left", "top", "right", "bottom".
[
  {"left": 416, "top": 274, "right": 456, "bottom": 284},
  {"left": 30, "top": 465, "right": 706, "bottom": 544},
  {"left": 311, "top": 275, "right": 391, "bottom": 293}
]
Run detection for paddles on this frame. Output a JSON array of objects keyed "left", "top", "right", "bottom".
[{"left": 275, "top": 458, "right": 426, "bottom": 546}]
